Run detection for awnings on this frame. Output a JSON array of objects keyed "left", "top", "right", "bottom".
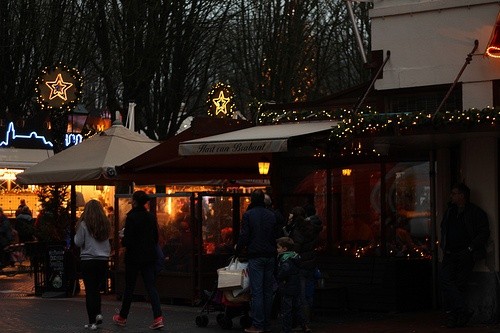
[
  {"left": 0, "top": 148, "right": 55, "bottom": 168},
  {"left": 116, "top": 122, "right": 273, "bottom": 176},
  {"left": 178, "top": 120, "right": 344, "bottom": 156}
]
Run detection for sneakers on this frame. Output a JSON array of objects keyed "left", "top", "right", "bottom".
[
  {"left": 96, "top": 315, "right": 104, "bottom": 325},
  {"left": 112, "top": 313, "right": 128, "bottom": 326},
  {"left": 151, "top": 317, "right": 165, "bottom": 331},
  {"left": 84, "top": 323, "right": 98, "bottom": 331},
  {"left": 244, "top": 322, "right": 272, "bottom": 333}
]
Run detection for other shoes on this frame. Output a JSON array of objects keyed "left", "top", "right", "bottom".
[
  {"left": 446, "top": 306, "right": 474, "bottom": 327},
  {"left": 292, "top": 322, "right": 312, "bottom": 333}
]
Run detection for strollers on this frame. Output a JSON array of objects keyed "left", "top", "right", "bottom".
[{"left": 195, "top": 240, "right": 251, "bottom": 329}]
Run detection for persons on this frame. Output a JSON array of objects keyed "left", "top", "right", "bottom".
[
  {"left": 112, "top": 191, "right": 165, "bottom": 330},
  {"left": 235, "top": 190, "right": 323, "bottom": 332},
  {"left": 74, "top": 200, "right": 111, "bottom": 330},
  {"left": 440, "top": 183, "right": 490, "bottom": 327},
  {"left": 0, "top": 200, "right": 37, "bottom": 268},
  {"left": 394, "top": 217, "right": 442, "bottom": 253}
]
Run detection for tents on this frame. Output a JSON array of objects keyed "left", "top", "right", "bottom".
[{"left": 15, "top": 124, "right": 162, "bottom": 258}]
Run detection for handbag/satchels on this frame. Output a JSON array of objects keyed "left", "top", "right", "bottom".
[{"left": 217, "top": 257, "right": 252, "bottom": 297}]
